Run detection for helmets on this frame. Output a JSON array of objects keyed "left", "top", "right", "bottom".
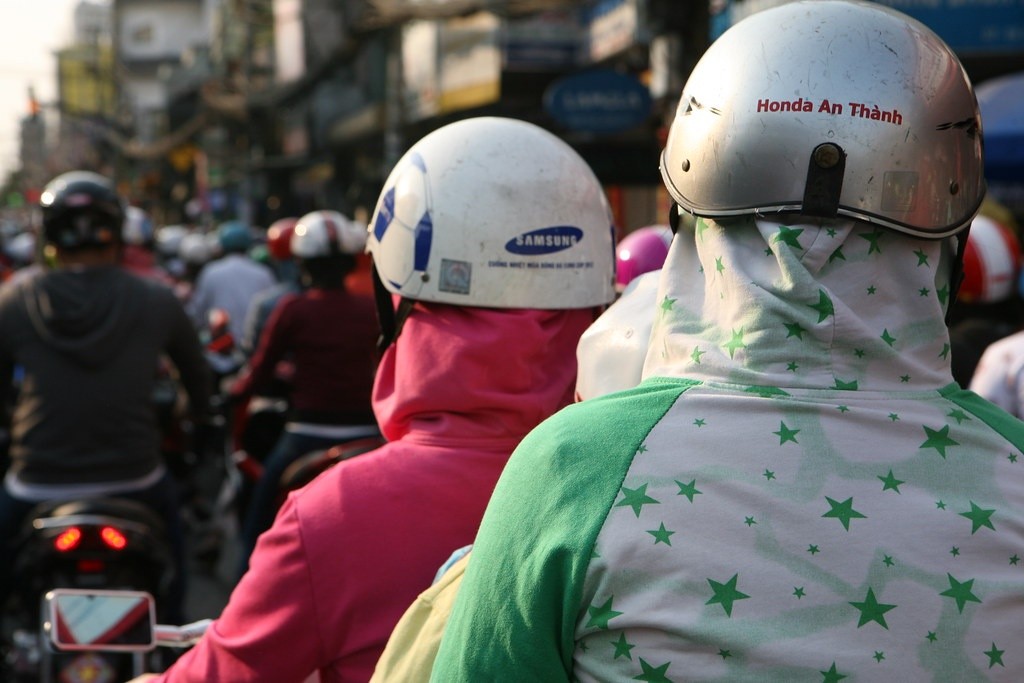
[
  {"left": 157, "top": 226, "right": 182, "bottom": 257},
  {"left": 659, "top": 0, "right": 989, "bottom": 242},
  {"left": 614, "top": 223, "right": 679, "bottom": 291},
  {"left": 38, "top": 172, "right": 124, "bottom": 249},
  {"left": 956, "top": 213, "right": 1022, "bottom": 306},
  {"left": 180, "top": 236, "right": 213, "bottom": 268},
  {"left": 290, "top": 214, "right": 362, "bottom": 262},
  {"left": 120, "top": 199, "right": 152, "bottom": 247},
  {"left": 364, "top": 117, "right": 616, "bottom": 311},
  {"left": 269, "top": 219, "right": 300, "bottom": 260}
]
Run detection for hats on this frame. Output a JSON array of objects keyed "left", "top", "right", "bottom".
[{"left": 219, "top": 223, "right": 251, "bottom": 254}]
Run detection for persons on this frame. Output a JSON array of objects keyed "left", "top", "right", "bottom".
[
  {"left": 368, "top": 0, "right": 1024, "bottom": 683},
  {"left": 0, "top": 80, "right": 1024, "bottom": 683}
]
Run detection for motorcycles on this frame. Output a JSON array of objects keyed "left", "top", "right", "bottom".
[{"left": 0, "top": 310, "right": 391, "bottom": 683}]
